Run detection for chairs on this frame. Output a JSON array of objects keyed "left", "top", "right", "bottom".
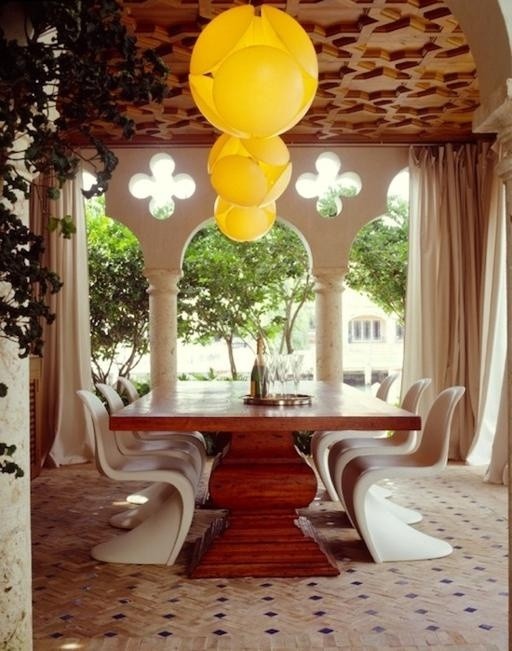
[
  {"left": 74, "top": 376, "right": 208, "bottom": 568},
  {"left": 312, "top": 372, "right": 467, "bottom": 565}
]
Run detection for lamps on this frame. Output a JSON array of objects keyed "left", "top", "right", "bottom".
[{"left": 188, "top": 2, "right": 318, "bottom": 244}]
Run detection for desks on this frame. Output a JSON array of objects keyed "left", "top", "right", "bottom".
[{"left": 108, "top": 379, "right": 422, "bottom": 579}]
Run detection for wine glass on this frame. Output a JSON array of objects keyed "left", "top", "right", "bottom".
[{"left": 254, "top": 352, "right": 305, "bottom": 401}]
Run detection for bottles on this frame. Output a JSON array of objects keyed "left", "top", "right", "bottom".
[{"left": 250, "top": 335, "right": 269, "bottom": 398}]
